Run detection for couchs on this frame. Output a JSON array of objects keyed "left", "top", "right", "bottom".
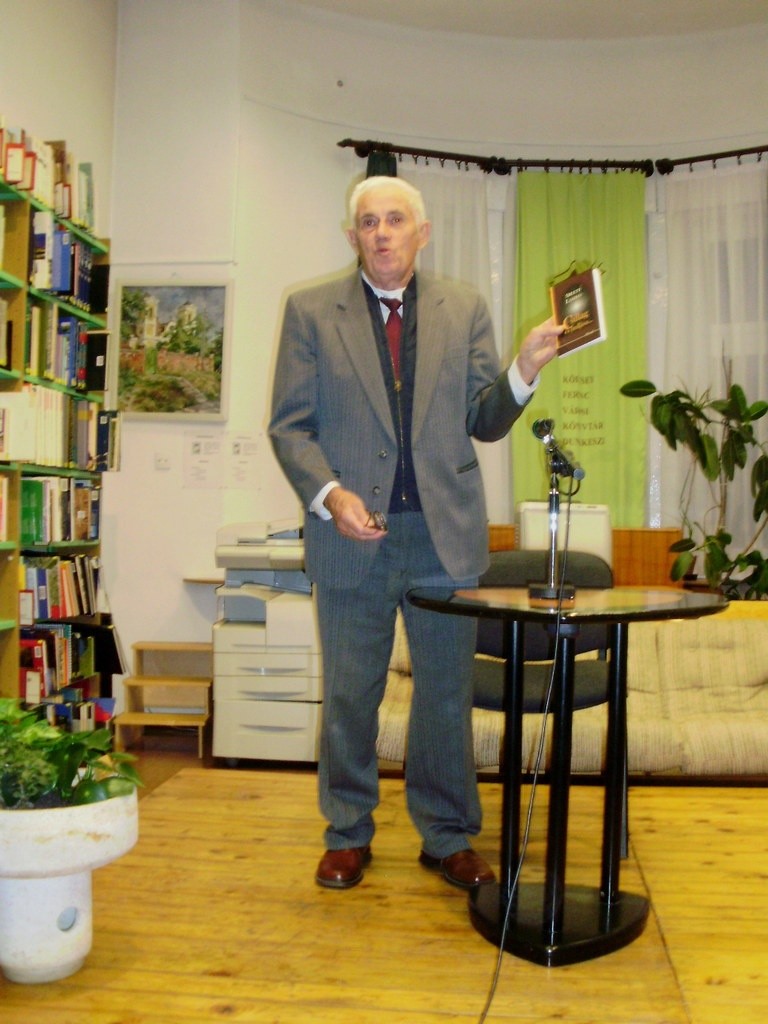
[{"left": 376, "top": 583, "right": 768, "bottom": 787}]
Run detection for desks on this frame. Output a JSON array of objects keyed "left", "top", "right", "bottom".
[{"left": 405, "top": 587, "right": 730, "bottom": 968}]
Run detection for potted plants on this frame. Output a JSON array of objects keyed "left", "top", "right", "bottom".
[{"left": 0, "top": 697, "right": 147, "bottom": 986}]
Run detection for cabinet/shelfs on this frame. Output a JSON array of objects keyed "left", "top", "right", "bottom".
[{"left": 0, "top": 161, "right": 110, "bottom": 726}]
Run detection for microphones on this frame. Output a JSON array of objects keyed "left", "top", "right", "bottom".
[{"left": 533, "top": 418, "right": 554, "bottom": 438}]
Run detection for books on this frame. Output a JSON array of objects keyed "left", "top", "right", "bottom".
[
  {"left": 1, "top": 128, "right": 127, "bottom": 733},
  {"left": 549, "top": 269, "right": 606, "bottom": 357}
]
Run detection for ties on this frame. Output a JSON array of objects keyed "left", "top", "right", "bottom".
[{"left": 379, "top": 297, "right": 402, "bottom": 384}]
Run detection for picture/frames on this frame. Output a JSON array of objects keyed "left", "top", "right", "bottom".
[{"left": 108, "top": 275, "right": 235, "bottom": 423}]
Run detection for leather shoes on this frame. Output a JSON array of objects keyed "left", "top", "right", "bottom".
[
  {"left": 419, "top": 848, "right": 497, "bottom": 889},
  {"left": 314, "top": 845, "right": 371, "bottom": 886}
]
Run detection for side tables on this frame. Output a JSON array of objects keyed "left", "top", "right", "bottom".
[{"left": 210, "top": 543, "right": 324, "bottom": 765}]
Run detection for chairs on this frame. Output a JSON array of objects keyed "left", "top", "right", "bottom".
[{"left": 473, "top": 547, "right": 628, "bottom": 860}]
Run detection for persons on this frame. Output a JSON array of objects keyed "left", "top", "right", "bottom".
[{"left": 269, "top": 177, "right": 563, "bottom": 889}]
